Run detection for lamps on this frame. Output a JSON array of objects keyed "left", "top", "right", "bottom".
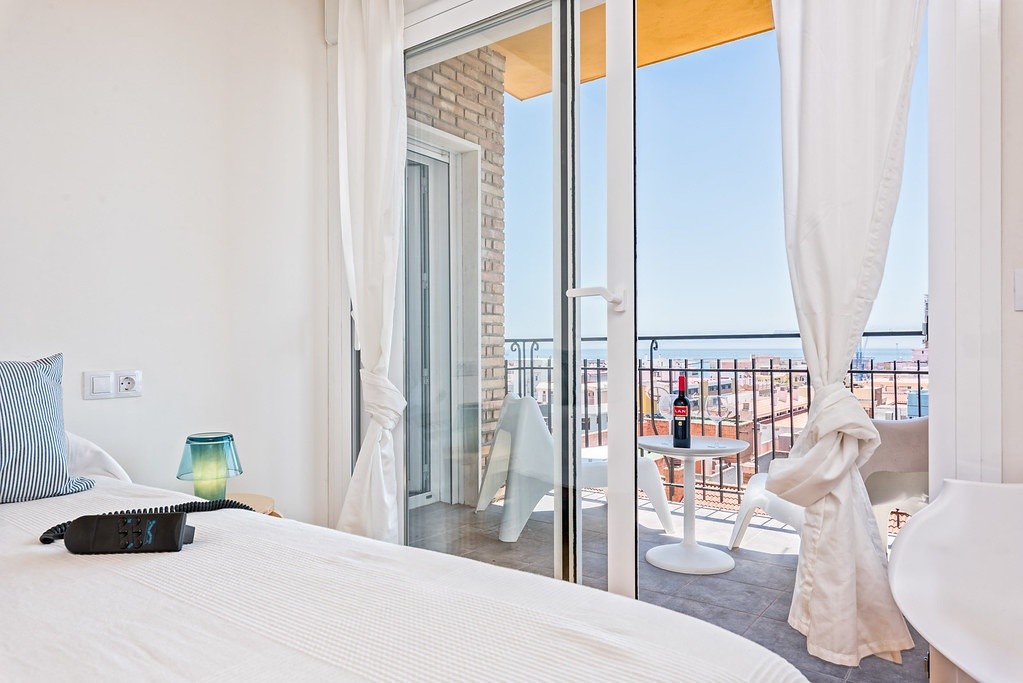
[{"left": 174, "top": 432, "right": 243, "bottom": 500}]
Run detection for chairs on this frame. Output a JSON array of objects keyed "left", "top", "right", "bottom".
[
  {"left": 727, "top": 415, "right": 929, "bottom": 551},
  {"left": 473, "top": 393, "right": 675, "bottom": 543}
]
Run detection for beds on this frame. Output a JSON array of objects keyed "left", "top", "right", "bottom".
[{"left": 0, "top": 428, "right": 810, "bottom": 683}]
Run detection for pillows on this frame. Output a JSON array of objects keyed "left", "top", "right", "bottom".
[{"left": 0, "top": 352, "right": 97, "bottom": 505}]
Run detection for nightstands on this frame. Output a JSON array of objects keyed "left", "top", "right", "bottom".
[{"left": 228, "top": 493, "right": 282, "bottom": 518}]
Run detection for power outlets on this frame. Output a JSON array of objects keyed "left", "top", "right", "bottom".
[{"left": 115, "top": 369, "right": 144, "bottom": 397}]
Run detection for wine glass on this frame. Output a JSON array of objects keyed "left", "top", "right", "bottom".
[
  {"left": 658, "top": 394, "right": 679, "bottom": 445},
  {"left": 705, "top": 395, "right": 730, "bottom": 447}
]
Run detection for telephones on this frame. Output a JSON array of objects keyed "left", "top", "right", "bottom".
[{"left": 63, "top": 512, "right": 195, "bottom": 553}]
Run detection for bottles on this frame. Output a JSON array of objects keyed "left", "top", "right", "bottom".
[{"left": 672, "top": 375, "right": 691, "bottom": 448}]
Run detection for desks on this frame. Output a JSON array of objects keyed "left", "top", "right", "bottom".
[{"left": 638, "top": 434, "right": 750, "bottom": 575}]
